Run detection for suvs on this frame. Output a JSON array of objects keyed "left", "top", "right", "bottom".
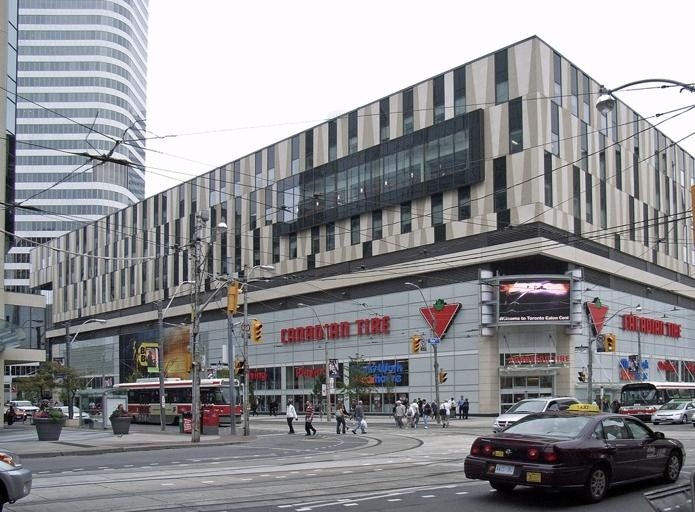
[{"left": 4, "top": 400, "right": 40, "bottom": 422}]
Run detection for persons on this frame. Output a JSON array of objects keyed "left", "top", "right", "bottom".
[
  {"left": 303, "top": 400, "right": 317, "bottom": 436},
  {"left": 318, "top": 399, "right": 325, "bottom": 422},
  {"left": 391, "top": 394, "right": 470, "bottom": 428},
  {"left": 335, "top": 400, "right": 350, "bottom": 435},
  {"left": 251, "top": 398, "right": 279, "bottom": 417},
  {"left": 285, "top": 398, "right": 299, "bottom": 434},
  {"left": 591, "top": 398, "right": 620, "bottom": 414},
  {"left": 350, "top": 400, "right": 368, "bottom": 434}
]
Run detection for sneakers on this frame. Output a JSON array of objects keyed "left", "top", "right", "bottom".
[
  {"left": 288, "top": 431, "right": 294, "bottom": 434},
  {"left": 362, "top": 431, "right": 367, "bottom": 434},
  {"left": 352, "top": 430, "right": 357, "bottom": 434},
  {"left": 305, "top": 430, "right": 316, "bottom": 436},
  {"left": 337, "top": 430, "right": 345, "bottom": 434}
]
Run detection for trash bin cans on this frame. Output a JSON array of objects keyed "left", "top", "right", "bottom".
[
  {"left": 182, "top": 411, "right": 192, "bottom": 434},
  {"left": 450, "top": 407, "right": 456, "bottom": 418}
]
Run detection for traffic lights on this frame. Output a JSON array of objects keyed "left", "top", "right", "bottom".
[
  {"left": 438, "top": 372, "right": 447, "bottom": 384},
  {"left": 412, "top": 335, "right": 422, "bottom": 353},
  {"left": 605, "top": 336, "right": 615, "bottom": 351},
  {"left": 251, "top": 321, "right": 263, "bottom": 343},
  {"left": 578, "top": 371, "right": 585, "bottom": 383}
]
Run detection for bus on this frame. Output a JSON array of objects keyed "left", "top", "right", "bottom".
[
  {"left": 112, "top": 377, "right": 243, "bottom": 427},
  {"left": 618, "top": 381, "right": 695, "bottom": 419}
]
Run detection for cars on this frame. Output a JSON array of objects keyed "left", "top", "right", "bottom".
[
  {"left": 53, "top": 406, "right": 91, "bottom": 423},
  {"left": 491, "top": 397, "right": 582, "bottom": 434},
  {"left": 651, "top": 398, "right": 695, "bottom": 424},
  {"left": 1, "top": 446, "right": 32, "bottom": 512},
  {"left": 464, "top": 403, "right": 686, "bottom": 503}
]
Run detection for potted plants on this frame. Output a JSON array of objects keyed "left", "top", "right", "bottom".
[
  {"left": 33, "top": 408, "right": 68, "bottom": 441},
  {"left": 108, "top": 406, "right": 134, "bottom": 434}
]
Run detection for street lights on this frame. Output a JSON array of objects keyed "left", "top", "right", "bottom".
[
  {"left": 297, "top": 303, "right": 335, "bottom": 420},
  {"left": 153, "top": 280, "right": 196, "bottom": 430},
  {"left": 66, "top": 318, "right": 107, "bottom": 418},
  {"left": 242, "top": 265, "right": 275, "bottom": 435},
  {"left": 596, "top": 78, "right": 695, "bottom": 115},
  {"left": 20, "top": 318, "right": 46, "bottom": 350},
  {"left": 192, "top": 223, "right": 228, "bottom": 443},
  {"left": 405, "top": 282, "right": 440, "bottom": 422},
  {"left": 585, "top": 304, "right": 642, "bottom": 403}
]
